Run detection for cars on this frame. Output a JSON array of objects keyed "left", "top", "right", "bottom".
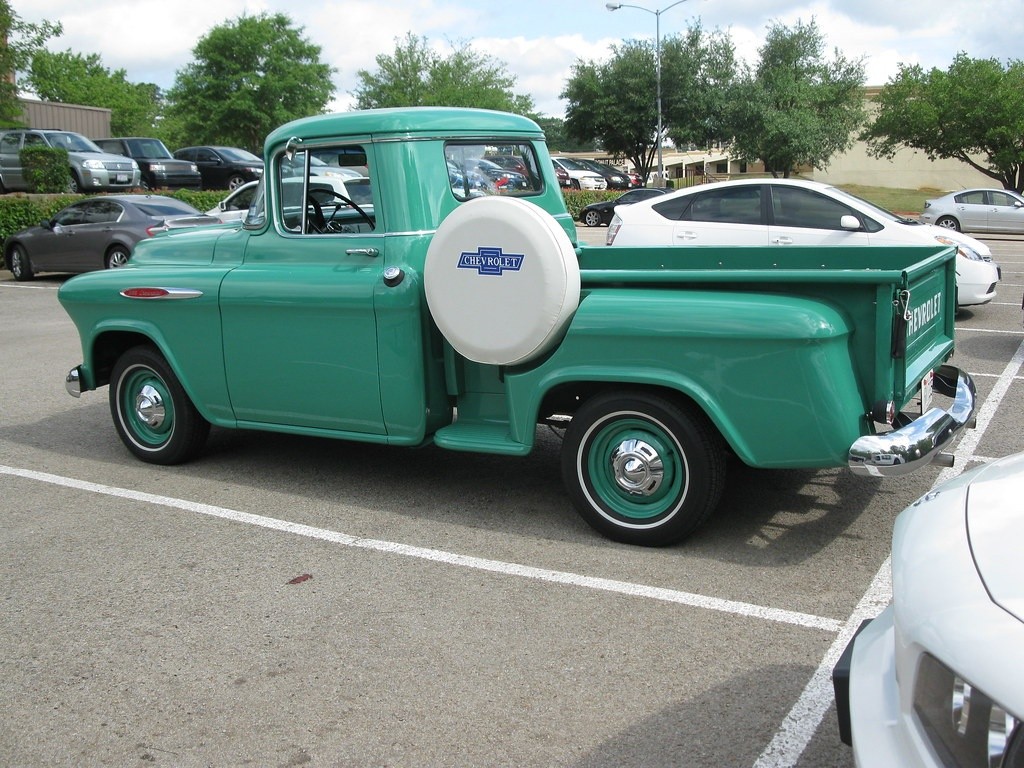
[
  {"left": 170, "top": 145, "right": 264, "bottom": 194},
  {"left": 274, "top": 153, "right": 364, "bottom": 185},
  {"left": 831, "top": 457, "right": 1023, "bottom": 768},
  {"left": 920, "top": 188, "right": 1024, "bottom": 235},
  {"left": 445, "top": 154, "right": 646, "bottom": 204},
  {"left": 322, "top": 150, "right": 370, "bottom": 179},
  {"left": 201, "top": 173, "right": 374, "bottom": 233},
  {"left": 605, "top": 177, "right": 1003, "bottom": 319},
  {"left": 4, "top": 192, "right": 226, "bottom": 282}
]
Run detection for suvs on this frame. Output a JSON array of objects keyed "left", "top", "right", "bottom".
[
  {"left": 86, "top": 136, "right": 204, "bottom": 195},
  {"left": 0, "top": 127, "right": 142, "bottom": 194}
]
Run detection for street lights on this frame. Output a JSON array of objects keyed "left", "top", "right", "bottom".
[{"left": 603, "top": 0, "right": 718, "bottom": 190}]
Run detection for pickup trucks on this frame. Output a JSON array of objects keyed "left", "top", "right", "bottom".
[{"left": 54, "top": 106, "right": 978, "bottom": 548}]
